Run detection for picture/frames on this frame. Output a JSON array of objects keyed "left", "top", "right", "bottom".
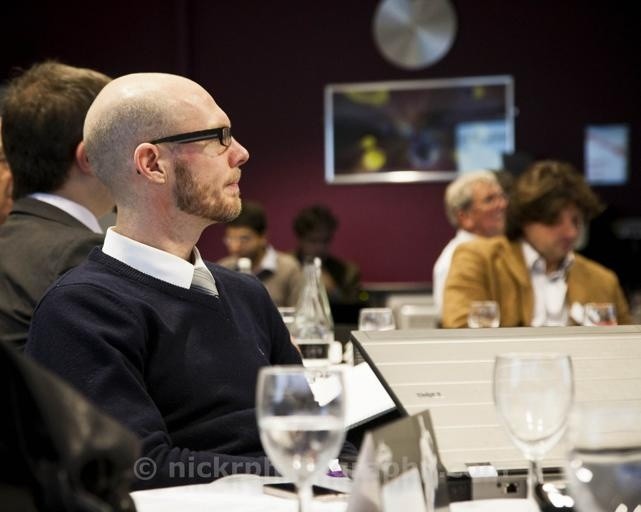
[{"left": 323, "top": 77, "right": 515, "bottom": 184}]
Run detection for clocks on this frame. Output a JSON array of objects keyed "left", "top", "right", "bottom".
[{"left": 373, "top": 1, "right": 458, "bottom": 71}]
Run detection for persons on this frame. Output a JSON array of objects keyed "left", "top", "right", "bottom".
[
  {"left": 214, "top": 200, "right": 304, "bottom": 312},
  {"left": 440, "top": 159, "right": 632, "bottom": 326},
  {"left": 289, "top": 205, "right": 373, "bottom": 325},
  {"left": 24, "top": 70, "right": 303, "bottom": 481},
  {"left": 431, "top": 169, "right": 509, "bottom": 319},
  {"left": 0, "top": 60, "right": 112, "bottom": 358}
]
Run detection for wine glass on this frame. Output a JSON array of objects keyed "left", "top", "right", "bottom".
[
  {"left": 255, "top": 365, "right": 346, "bottom": 512},
  {"left": 544, "top": 304, "right": 568, "bottom": 326},
  {"left": 582, "top": 302, "right": 618, "bottom": 326},
  {"left": 358, "top": 308, "right": 395, "bottom": 331},
  {"left": 466, "top": 299, "right": 501, "bottom": 327},
  {"left": 494, "top": 352, "right": 573, "bottom": 512}
]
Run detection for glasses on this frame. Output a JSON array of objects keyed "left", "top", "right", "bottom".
[{"left": 136, "top": 128, "right": 232, "bottom": 173}]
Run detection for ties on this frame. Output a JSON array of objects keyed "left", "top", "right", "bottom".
[
  {"left": 190, "top": 269, "right": 218, "bottom": 297},
  {"left": 239, "top": 258, "right": 250, "bottom": 273}
]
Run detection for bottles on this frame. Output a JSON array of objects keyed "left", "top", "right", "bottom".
[{"left": 291, "top": 265, "right": 334, "bottom": 341}]
